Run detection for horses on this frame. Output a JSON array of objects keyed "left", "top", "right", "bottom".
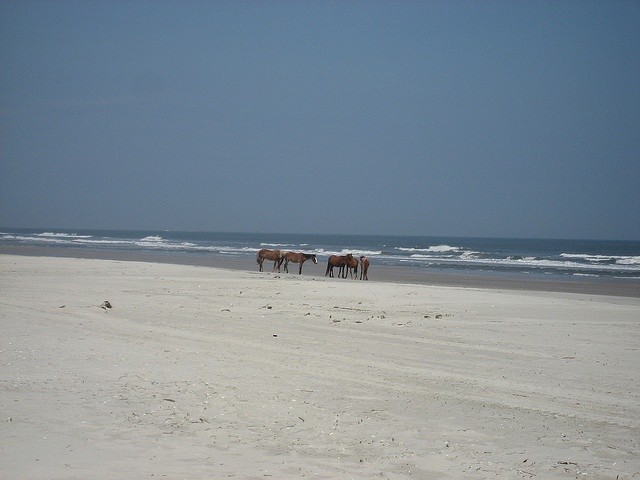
[
  {"left": 360, "top": 256, "right": 369, "bottom": 280},
  {"left": 324, "top": 253, "right": 354, "bottom": 279},
  {"left": 338, "top": 256, "right": 358, "bottom": 280},
  {"left": 275, "top": 251, "right": 318, "bottom": 275},
  {"left": 257, "top": 249, "right": 290, "bottom": 273}
]
